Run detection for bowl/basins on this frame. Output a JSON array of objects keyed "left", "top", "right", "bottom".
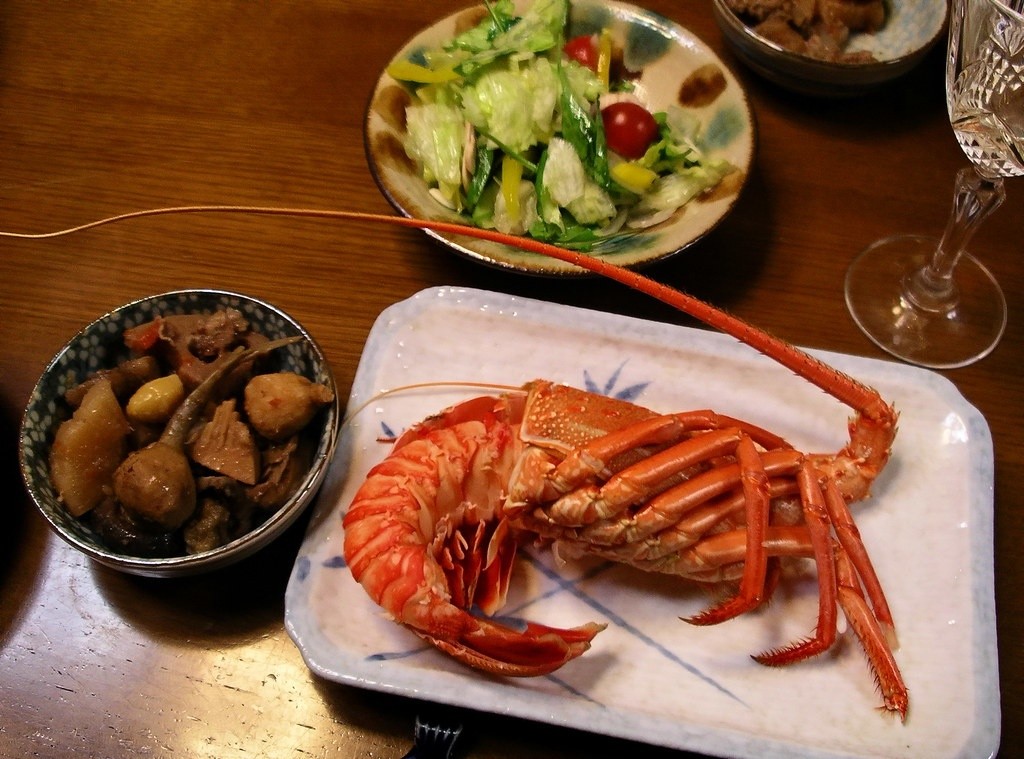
[
  {"left": 20, "top": 289, "right": 340, "bottom": 579},
  {"left": 713, "top": 0, "right": 950, "bottom": 74}
]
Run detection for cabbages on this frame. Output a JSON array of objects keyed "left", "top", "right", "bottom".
[{"left": 384, "top": 0, "right": 735, "bottom": 248}]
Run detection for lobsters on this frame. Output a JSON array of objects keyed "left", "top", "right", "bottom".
[{"left": 0, "top": 203, "right": 910, "bottom": 728}]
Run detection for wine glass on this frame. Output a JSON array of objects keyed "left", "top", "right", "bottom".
[{"left": 843, "top": 0, "right": 1024, "bottom": 368}]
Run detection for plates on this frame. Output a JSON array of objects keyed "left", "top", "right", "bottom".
[
  {"left": 367, "top": 0, "right": 756, "bottom": 282},
  {"left": 283, "top": 286, "right": 1001, "bottom": 759}
]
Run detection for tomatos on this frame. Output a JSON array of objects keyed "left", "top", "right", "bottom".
[
  {"left": 563, "top": 35, "right": 597, "bottom": 72},
  {"left": 598, "top": 102, "right": 659, "bottom": 159}
]
